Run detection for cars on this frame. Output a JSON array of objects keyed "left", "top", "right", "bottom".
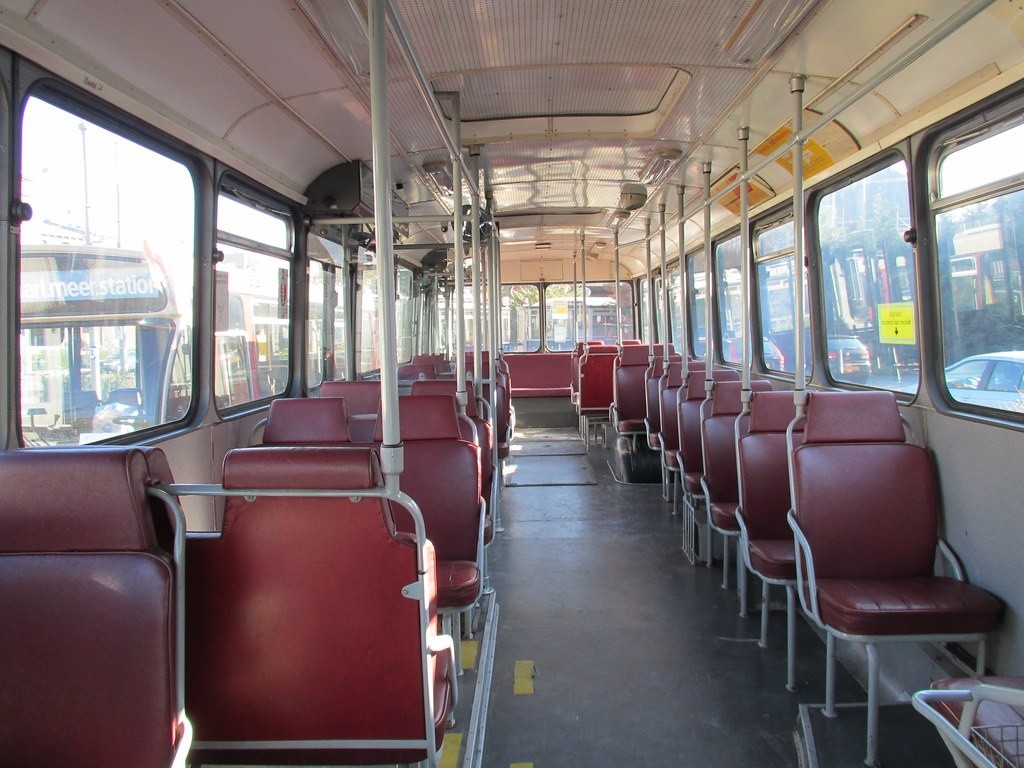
[
  {"left": 699, "top": 335, "right": 784, "bottom": 372},
  {"left": 900, "top": 350, "right": 1024, "bottom": 414}
]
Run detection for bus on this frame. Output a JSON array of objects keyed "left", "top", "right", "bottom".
[{"left": 22, "top": 242, "right": 367, "bottom": 446}]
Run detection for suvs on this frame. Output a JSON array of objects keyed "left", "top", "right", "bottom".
[{"left": 785, "top": 335, "right": 873, "bottom": 383}]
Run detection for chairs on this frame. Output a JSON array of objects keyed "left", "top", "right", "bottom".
[
  {"left": 911, "top": 674, "right": 1024, "bottom": 768},
  {"left": 0, "top": 349, "right": 514, "bottom": 768},
  {"left": 735, "top": 389, "right": 851, "bottom": 647},
  {"left": 787, "top": 390, "right": 1002, "bottom": 768},
  {"left": 571, "top": 339, "right": 779, "bottom": 590}
]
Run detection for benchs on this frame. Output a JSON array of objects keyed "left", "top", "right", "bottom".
[{"left": 499, "top": 351, "right": 580, "bottom": 428}]
